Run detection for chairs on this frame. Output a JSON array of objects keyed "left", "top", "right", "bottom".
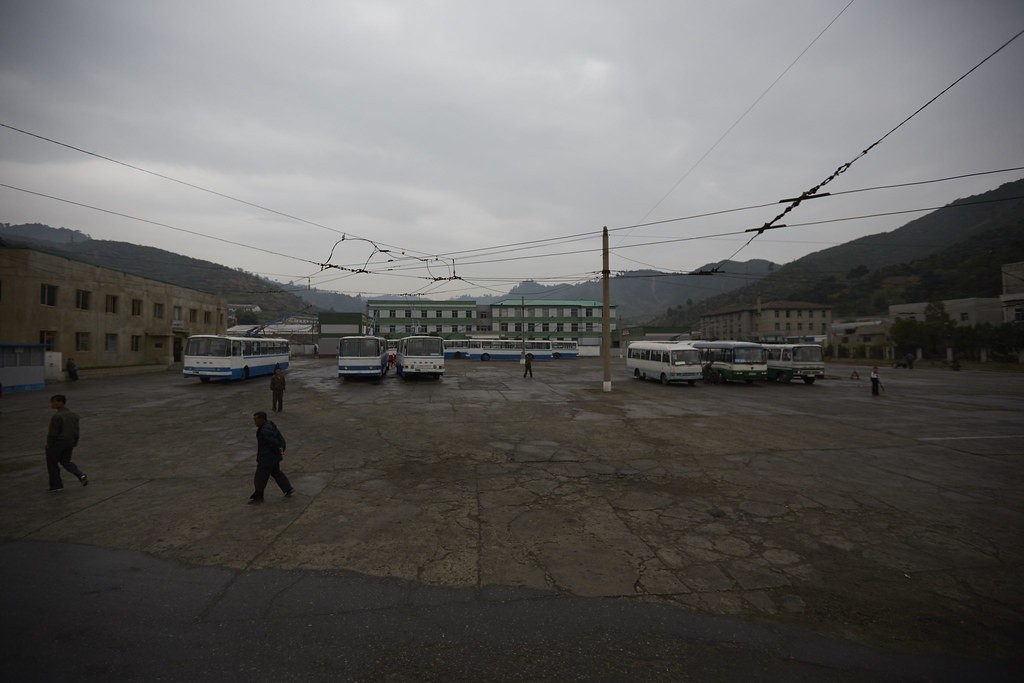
[
  {"left": 243, "top": 346, "right": 287, "bottom": 355},
  {"left": 701, "top": 353, "right": 732, "bottom": 362},
  {"left": 635, "top": 353, "right": 669, "bottom": 362}
]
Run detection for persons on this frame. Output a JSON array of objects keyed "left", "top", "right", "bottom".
[
  {"left": 246, "top": 411, "right": 296, "bottom": 507},
  {"left": 45, "top": 394, "right": 89, "bottom": 495},
  {"left": 523, "top": 354, "right": 533, "bottom": 378},
  {"left": 870, "top": 367, "right": 879, "bottom": 397},
  {"left": 270, "top": 367, "right": 286, "bottom": 412}
]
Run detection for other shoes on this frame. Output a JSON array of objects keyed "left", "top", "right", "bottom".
[
  {"left": 284, "top": 489, "right": 295, "bottom": 497},
  {"left": 249, "top": 495, "right": 264, "bottom": 500},
  {"left": 47, "top": 485, "right": 63, "bottom": 492},
  {"left": 79, "top": 474, "right": 88, "bottom": 487}
]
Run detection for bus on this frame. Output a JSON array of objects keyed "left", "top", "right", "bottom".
[
  {"left": 549, "top": 340, "right": 580, "bottom": 359},
  {"left": 336, "top": 335, "right": 391, "bottom": 380},
  {"left": 395, "top": 334, "right": 448, "bottom": 381},
  {"left": 466, "top": 339, "right": 554, "bottom": 361},
  {"left": 760, "top": 343, "right": 828, "bottom": 383},
  {"left": 442, "top": 340, "right": 470, "bottom": 359},
  {"left": 386, "top": 339, "right": 398, "bottom": 361},
  {"left": 688, "top": 340, "right": 771, "bottom": 384},
  {"left": 626, "top": 342, "right": 705, "bottom": 386},
  {"left": 181, "top": 335, "right": 291, "bottom": 383}
]
